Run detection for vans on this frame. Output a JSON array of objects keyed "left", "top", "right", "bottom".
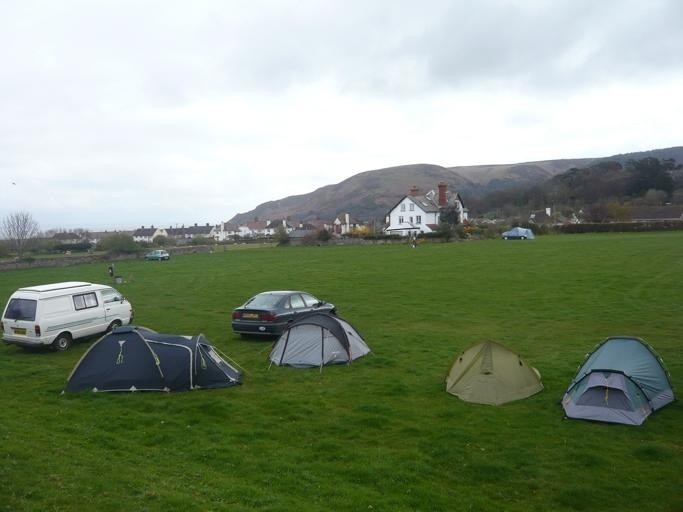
[{"left": 0, "top": 281, "right": 134, "bottom": 352}]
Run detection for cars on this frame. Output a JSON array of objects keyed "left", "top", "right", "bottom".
[
  {"left": 232, "top": 290, "right": 337, "bottom": 340},
  {"left": 502, "top": 231, "right": 528, "bottom": 240},
  {"left": 145, "top": 250, "right": 170, "bottom": 261}
]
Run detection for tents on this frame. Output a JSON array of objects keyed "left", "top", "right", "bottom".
[
  {"left": 58, "top": 324, "right": 244, "bottom": 399},
  {"left": 560, "top": 336, "right": 676, "bottom": 427},
  {"left": 265, "top": 311, "right": 371, "bottom": 370},
  {"left": 444, "top": 338, "right": 545, "bottom": 407}
]
